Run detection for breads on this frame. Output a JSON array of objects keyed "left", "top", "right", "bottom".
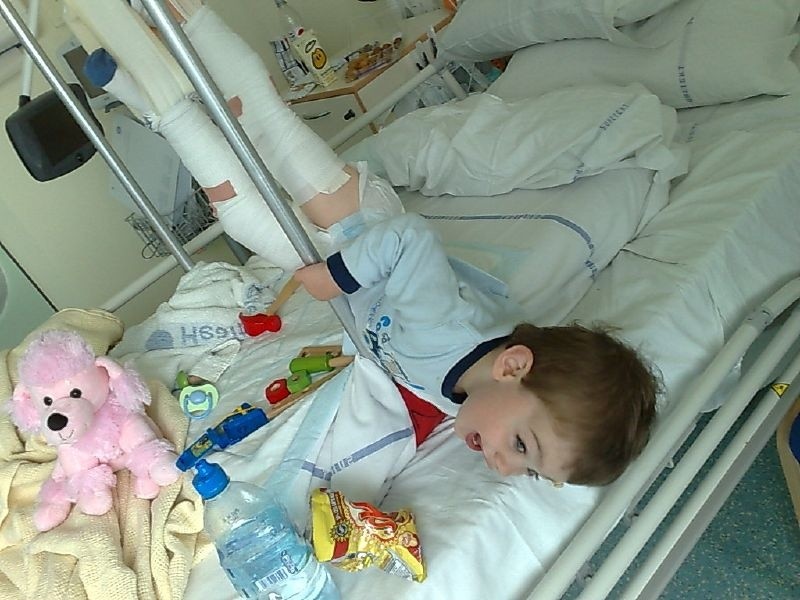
[{"left": 344, "top": 43, "right": 392, "bottom": 83}]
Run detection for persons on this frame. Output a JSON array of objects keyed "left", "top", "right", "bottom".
[{"left": 85, "top": 0, "right": 669, "bottom": 563}]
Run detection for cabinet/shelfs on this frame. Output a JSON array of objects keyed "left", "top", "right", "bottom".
[{"left": 276, "top": 8, "right": 450, "bottom": 154}]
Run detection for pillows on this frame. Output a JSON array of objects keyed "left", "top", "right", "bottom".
[
  {"left": 438, "top": 0, "right": 665, "bottom": 54},
  {"left": 470, "top": 0, "right": 800, "bottom": 101}
]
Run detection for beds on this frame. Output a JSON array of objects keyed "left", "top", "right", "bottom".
[{"left": 0, "top": 94, "right": 800, "bottom": 600}]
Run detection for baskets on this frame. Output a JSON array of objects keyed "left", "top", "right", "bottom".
[{"left": 126, "top": 183, "right": 217, "bottom": 257}]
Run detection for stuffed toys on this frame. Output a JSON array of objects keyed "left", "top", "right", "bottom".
[{"left": 10, "top": 329, "right": 182, "bottom": 531}]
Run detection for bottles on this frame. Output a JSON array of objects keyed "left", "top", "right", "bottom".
[
  {"left": 192, "top": 460, "right": 342, "bottom": 600},
  {"left": 274, "top": 0, "right": 338, "bottom": 89}
]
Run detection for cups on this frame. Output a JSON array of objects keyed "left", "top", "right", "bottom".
[{"left": 268, "top": 28, "right": 313, "bottom": 92}]
